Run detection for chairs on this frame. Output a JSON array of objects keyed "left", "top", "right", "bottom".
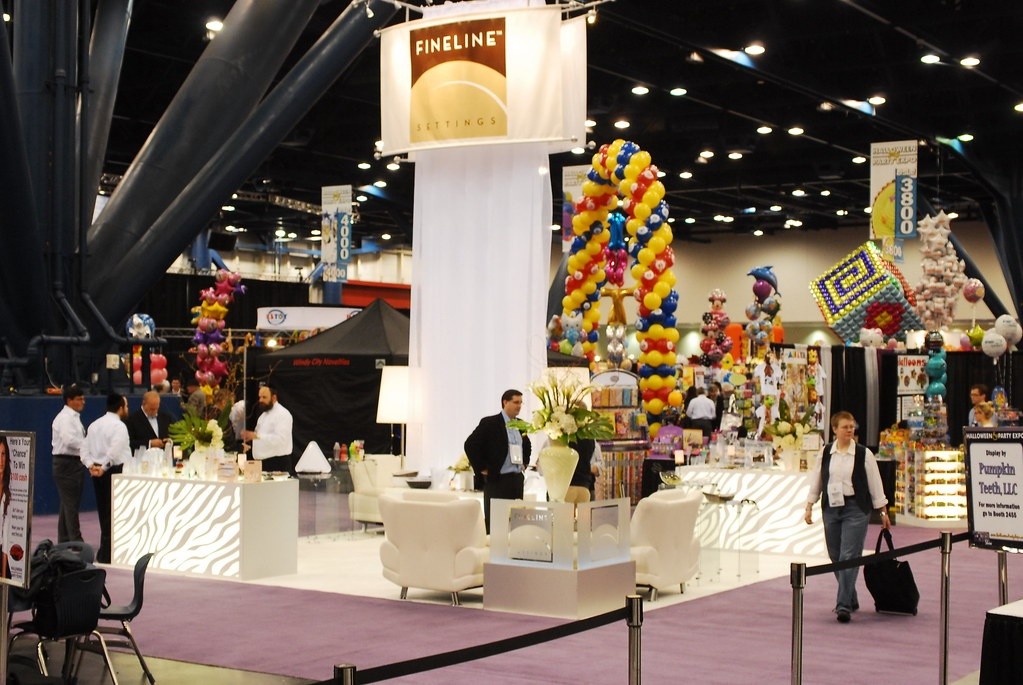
[{"left": 7, "top": 541, "right": 156, "bottom": 685}]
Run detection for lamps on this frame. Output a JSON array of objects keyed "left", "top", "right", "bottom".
[
  {"left": 376, "top": 365, "right": 417, "bottom": 470},
  {"left": 365, "top": 0, "right": 374, "bottom": 19}
]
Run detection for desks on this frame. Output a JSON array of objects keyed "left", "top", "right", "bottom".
[{"left": 110, "top": 473, "right": 300, "bottom": 581}]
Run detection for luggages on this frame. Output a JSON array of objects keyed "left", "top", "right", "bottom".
[{"left": 863, "top": 528, "right": 919, "bottom": 616}]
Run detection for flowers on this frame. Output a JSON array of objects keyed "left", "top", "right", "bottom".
[
  {"left": 505, "top": 368, "right": 614, "bottom": 443},
  {"left": 168, "top": 399, "right": 231, "bottom": 450},
  {"left": 763, "top": 396, "right": 815, "bottom": 451}
]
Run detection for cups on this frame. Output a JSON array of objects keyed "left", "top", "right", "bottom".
[
  {"left": 238, "top": 453, "right": 247, "bottom": 477},
  {"left": 123, "top": 438, "right": 175, "bottom": 479}
]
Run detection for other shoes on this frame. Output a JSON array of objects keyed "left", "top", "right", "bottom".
[
  {"left": 852, "top": 602, "right": 859, "bottom": 611},
  {"left": 837, "top": 610, "right": 850, "bottom": 623}
]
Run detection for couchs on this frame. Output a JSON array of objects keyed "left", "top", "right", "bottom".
[
  {"left": 628, "top": 488, "right": 702, "bottom": 601},
  {"left": 347, "top": 459, "right": 411, "bottom": 532},
  {"left": 379, "top": 487, "right": 490, "bottom": 607}
]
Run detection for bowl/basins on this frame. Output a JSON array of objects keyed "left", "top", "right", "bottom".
[{"left": 407, "top": 480, "right": 431, "bottom": 488}]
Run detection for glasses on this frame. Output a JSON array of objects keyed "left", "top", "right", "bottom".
[{"left": 507, "top": 401, "right": 522, "bottom": 404}]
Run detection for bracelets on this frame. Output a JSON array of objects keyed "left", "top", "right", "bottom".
[{"left": 880, "top": 512, "right": 887, "bottom": 517}]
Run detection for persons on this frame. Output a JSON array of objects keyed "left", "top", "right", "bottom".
[
  {"left": 184, "top": 378, "right": 200, "bottom": 395},
  {"left": 805, "top": 411, "right": 892, "bottom": 622},
  {"left": 169, "top": 378, "right": 188, "bottom": 404},
  {"left": 200, "top": 390, "right": 251, "bottom": 454},
  {"left": 683, "top": 381, "right": 724, "bottom": 436},
  {"left": 464, "top": 389, "right": 531, "bottom": 535},
  {"left": 547, "top": 398, "right": 596, "bottom": 505},
  {"left": 240, "top": 378, "right": 293, "bottom": 471},
  {"left": 124, "top": 390, "right": 177, "bottom": 457},
  {"left": 968, "top": 383, "right": 997, "bottom": 427},
  {"left": 640, "top": 409, "right": 683, "bottom": 500},
  {"left": 51, "top": 387, "right": 86, "bottom": 551},
  {"left": 0, "top": 436, "right": 11, "bottom": 582},
  {"left": 80, "top": 391, "right": 132, "bottom": 565},
  {"left": 230, "top": 399, "right": 246, "bottom": 440}
]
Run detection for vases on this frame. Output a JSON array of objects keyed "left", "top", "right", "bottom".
[
  {"left": 539, "top": 433, "right": 579, "bottom": 501},
  {"left": 782, "top": 450, "right": 801, "bottom": 474},
  {"left": 196, "top": 445, "right": 217, "bottom": 480}
]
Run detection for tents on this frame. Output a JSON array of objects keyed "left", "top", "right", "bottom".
[{"left": 254, "top": 297, "right": 410, "bottom": 475}]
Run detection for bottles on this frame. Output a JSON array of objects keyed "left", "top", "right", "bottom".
[
  {"left": 333, "top": 440, "right": 364, "bottom": 462},
  {"left": 708, "top": 430, "right": 772, "bottom": 469}
]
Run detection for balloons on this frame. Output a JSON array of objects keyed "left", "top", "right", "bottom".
[
  {"left": 547, "top": 140, "right": 682, "bottom": 438},
  {"left": 120, "top": 313, "right": 168, "bottom": 385},
  {"left": 964, "top": 278, "right": 985, "bottom": 304},
  {"left": 744, "top": 264, "right": 782, "bottom": 345},
  {"left": 961, "top": 314, "right": 1022, "bottom": 365},
  {"left": 915, "top": 210, "right": 969, "bottom": 402},
  {"left": 188, "top": 268, "right": 247, "bottom": 406},
  {"left": 992, "top": 386, "right": 1007, "bottom": 409},
  {"left": 808, "top": 239, "right": 926, "bottom": 344},
  {"left": 700, "top": 288, "right": 733, "bottom": 369}
]
